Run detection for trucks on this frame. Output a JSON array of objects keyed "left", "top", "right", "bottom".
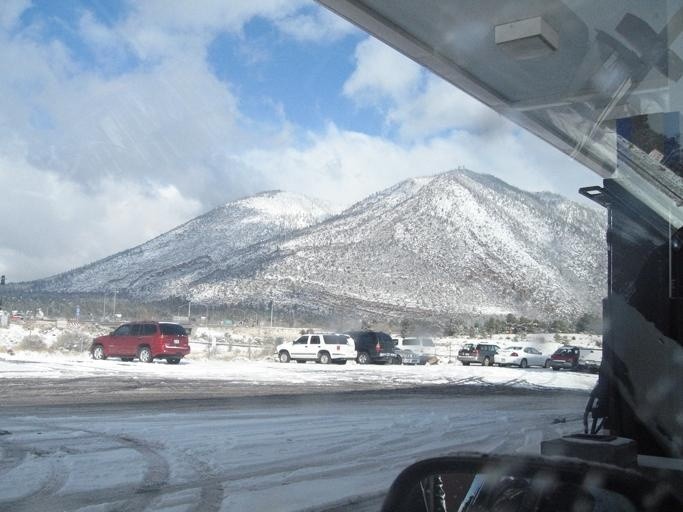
[{"left": 549, "top": 345, "right": 602, "bottom": 372}]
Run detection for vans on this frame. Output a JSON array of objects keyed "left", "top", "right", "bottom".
[{"left": 456, "top": 343, "right": 501, "bottom": 366}]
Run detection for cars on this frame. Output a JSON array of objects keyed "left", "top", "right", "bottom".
[{"left": 493, "top": 346, "right": 550, "bottom": 369}]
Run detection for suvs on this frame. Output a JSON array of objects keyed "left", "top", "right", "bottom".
[
  {"left": 90, "top": 322, "right": 189, "bottom": 363},
  {"left": 276, "top": 331, "right": 436, "bottom": 365}
]
